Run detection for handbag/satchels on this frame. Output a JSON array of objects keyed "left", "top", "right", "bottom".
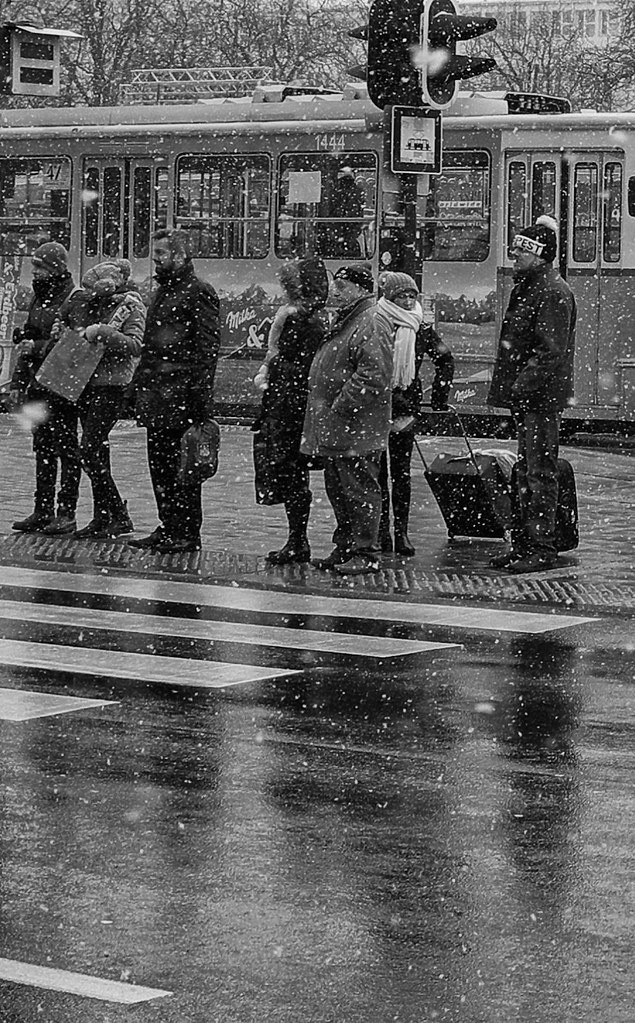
[
  {"left": 34, "top": 328, "right": 105, "bottom": 403},
  {"left": 510, "top": 456, "right": 579, "bottom": 553},
  {"left": 252, "top": 426, "right": 289, "bottom": 505},
  {"left": 177, "top": 418, "right": 221, "bottom": 487}
]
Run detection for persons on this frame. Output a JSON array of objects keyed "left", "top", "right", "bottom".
[
  {"left": 50, "top": 259, "right": 147, "bottom": 539},
  {"left": 121, "top": 229, "right": 221, "bottom": 554},
  {"left": 250, "top": 255, "right": 329, "bottom": 565},
  {"left": 375, "top": 270, "right": 455, "bottom": 557},
  {"left": 485, "top": 215, "right": 578, "bottom": 573},
  {"left": 299, "top": 262, "right": 393, "bottom": 576},
  {"left": 12, "top": 243, "right": 77, "bottom": 534}
]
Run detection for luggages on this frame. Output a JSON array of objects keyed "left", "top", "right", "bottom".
[{"left": 408, "top": 402, "right": 519, "bottom": 548}]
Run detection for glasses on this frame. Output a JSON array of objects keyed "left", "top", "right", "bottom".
[{"left": 398, "top": 293, "right": 416, "bottom": 300}]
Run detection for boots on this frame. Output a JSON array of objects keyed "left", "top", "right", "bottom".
[{"left": 73, "top": 500, "right": 134, "bottom": 540}]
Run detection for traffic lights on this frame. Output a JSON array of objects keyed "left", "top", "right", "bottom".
[
  {"left": 407, "top": 0, "right": 496, "bottom": 113},
  {"left": 347, "top": 0, "right": 424, "bottom": 113}
]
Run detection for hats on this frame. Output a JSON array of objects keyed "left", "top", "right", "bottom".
[
  {"left": 333, "top": 261, "right": 375, "bottom": 293},
  {"left": 378, "top": 270, "right": 420, "bottom": 300},
  {"left": 31, "top": 242, "right": 69, "bottom": 276},
  {"left": 512, "top": 214, "right": 558, "bottom": 263},
  {"left": 81, "top": 258, "right": 132, "bottom": 291}
]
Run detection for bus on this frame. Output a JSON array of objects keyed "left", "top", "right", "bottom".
[{"left": 0, "top": 67, "right": 635, "bottom": 423}]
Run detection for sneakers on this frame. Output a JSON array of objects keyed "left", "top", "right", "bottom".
[{"left": 126, "top": 526, "right": 203, "bottom": 555}]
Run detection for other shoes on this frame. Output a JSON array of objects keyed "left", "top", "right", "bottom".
[
  {"left": 37, "top": 515, "right": 77, "bottom": 536},
  {"left": 381, "top": 537, "right": 393, "bottom": 553},
  {"left": 488, "top": 552, "right": 558, "bottom": 573},
  {"left": 11, "top": 513, "right": 55, "bottom": 531},
  {"left": 395, "top": 536, "right": 415, "bottom": 557},
  {"left": 312, "top": 552, "right": 351, "bottom": 571},
  {"left": 265, "top": 542, "right": 311, "bottom": 565},
  {"left": 334, "top": 557, "right": 381, "bottom": 576}
]
